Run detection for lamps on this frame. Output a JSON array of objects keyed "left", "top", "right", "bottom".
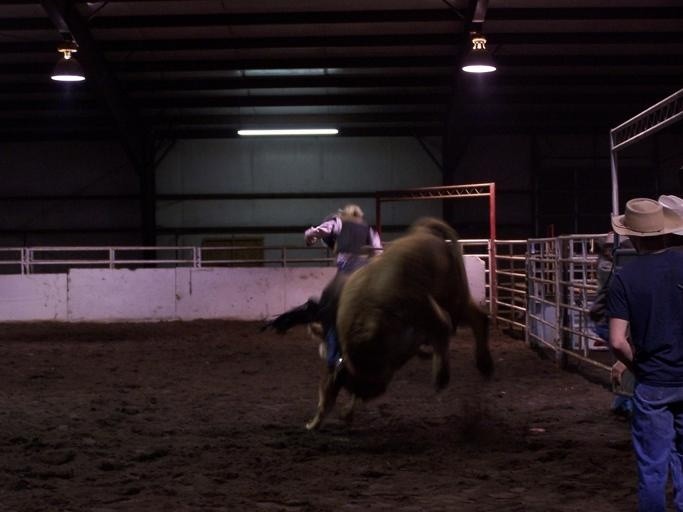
[
  {"left": 235, "top": 70, "right": 340, "bottom": 138},
  {"left": 50, "top": 18, "right": 86, "bottom": 82},
  {"left": 461, "top": 0, "right": 497, "bottom": 74}
]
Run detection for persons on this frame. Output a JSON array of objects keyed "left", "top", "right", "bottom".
[
  {"left": 588, "top": 191, "right": 683, "bottom": 387},
  {"left": 602, "top": 196, "right": 682, "bottom": 510},
  {"left": 301, "top": 202, "right": 387, "bottom": 373}
]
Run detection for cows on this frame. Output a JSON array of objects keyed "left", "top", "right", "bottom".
[{"left": 303, "top": 216, "right": 494, "bottom": 432}]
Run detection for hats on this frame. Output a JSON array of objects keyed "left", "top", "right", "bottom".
[{"left": 610, "top": 195, "right": 683, "bottom": 236}]
